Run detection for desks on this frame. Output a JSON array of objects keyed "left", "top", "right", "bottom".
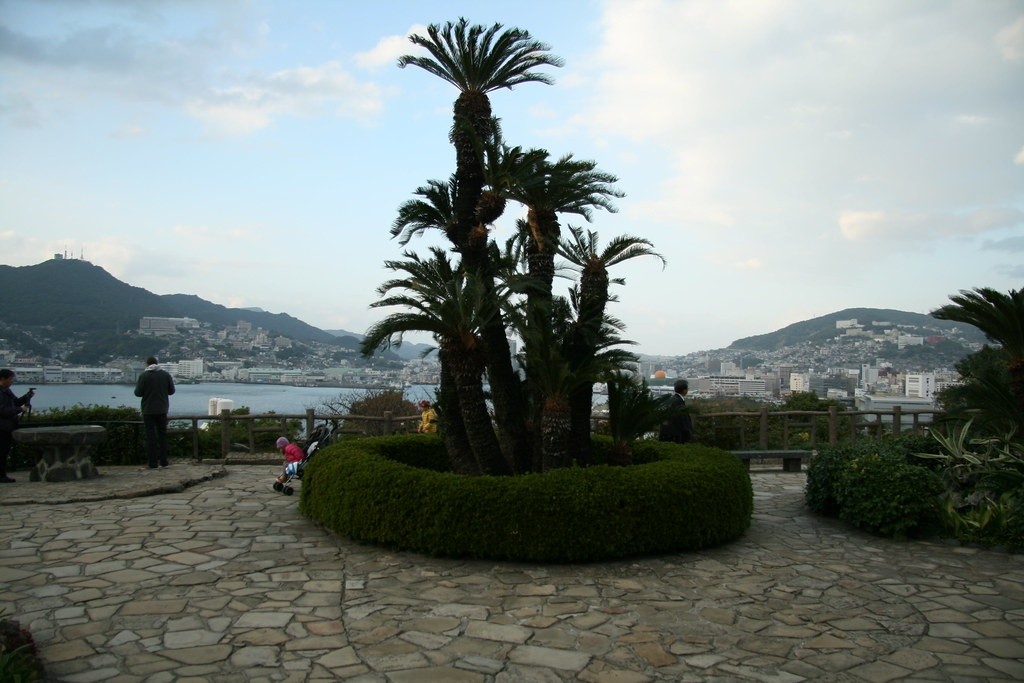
[{"left": 12, "top": 425, "right": 108, "bottom": 483}]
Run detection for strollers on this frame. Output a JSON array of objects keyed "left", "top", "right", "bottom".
[{"left": 274, "top": 419, "right": 338, "bottom": 496}]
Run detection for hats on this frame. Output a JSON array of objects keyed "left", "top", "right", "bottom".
[
  {"left": 277, "top": 437, "right": 289, "bottom": 448},
  {"left": 418, "top": 399, "right": 430, "bottom": 407}
]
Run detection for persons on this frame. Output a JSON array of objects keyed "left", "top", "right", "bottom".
[
  {"left": 134, "top": 357, "right": 175, "bottom": 468},
  {"left": 276, "top": 437, "right": 305, "bottom": 482},
  {"left": 0, "top": 369, "right": 35, "bottom": 484},
  {"left": 659, "top": 380, "right": 695, "bottom": 442},
  {"left": 418, "top": 400, "right": 438, "bottom": 433}
]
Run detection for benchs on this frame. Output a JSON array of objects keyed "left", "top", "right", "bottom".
[{"left": 728, "top": 450, "right": 811, "bottom": 472}]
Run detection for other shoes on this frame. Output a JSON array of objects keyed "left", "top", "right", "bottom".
[
  {"left": 143, "top": 464, "right": 160, "bottom": 471},
  {"left": 276, "top": 474, "right": 287, "bottom": 483},
  {"left": 159, "top": 465, "right": 169, "bottom": 469},
  {"left": 0, "top": 476, "right": 15, "bottom": 482}
]
